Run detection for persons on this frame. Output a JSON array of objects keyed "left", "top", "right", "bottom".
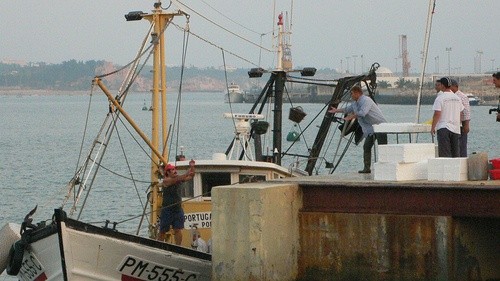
[
  {"left": 191, "top": 230, "right": 208, "bottom": 254},
  {"left": 431, "top": 76, "right": 471, "bottom": 158},
  {"left": 328, "top": 86, "right": 388, "bottom": 174},
  {"left": 492, "top": 72, "right": 500, "bottom": 122},
  {"left": 158, "top": 160, "right": 195, "bottom": 245}
]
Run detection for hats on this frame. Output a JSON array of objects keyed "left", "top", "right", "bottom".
[
  {"left": 164, "top": 164, "right": 175, "bottom": 171},
  {"left": 437, "top": 75, "right": 452, "bottom": 86}
]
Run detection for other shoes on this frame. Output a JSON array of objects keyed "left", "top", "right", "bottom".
[{"left": 358, "top": 168, "right": 371, "bottom": 173}]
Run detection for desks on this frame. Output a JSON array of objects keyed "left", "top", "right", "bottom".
[{"left": 373, "top": 131, "right": 436, "bottom": 163}]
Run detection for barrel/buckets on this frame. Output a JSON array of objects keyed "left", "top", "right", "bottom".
[
  {"left": 288, "top": 106, "right": 307, "bottom": 123},
  {"left": 467, "top": 150, "right": 488, "bottom": 181}
]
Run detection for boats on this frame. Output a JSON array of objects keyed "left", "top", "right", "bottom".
[
  {"left": 1, "top": 1, "right": 216, "bottom": 281},
  {"left": 147, "top": 0, "right": 500, "bottom": 281},
  {"left": 466, "top": 92, "right": 481, "bottom": 107},
  {"left": 224, "top": 81, "right": 243, "bottom": 104}
]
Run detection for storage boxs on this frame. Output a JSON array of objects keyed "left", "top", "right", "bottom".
[
  {"left": 372, "top": 144, "right": 468, "bottom": 182},
  {"left": 372, "top": 123, "right": 432, "bottom": 132}
]
